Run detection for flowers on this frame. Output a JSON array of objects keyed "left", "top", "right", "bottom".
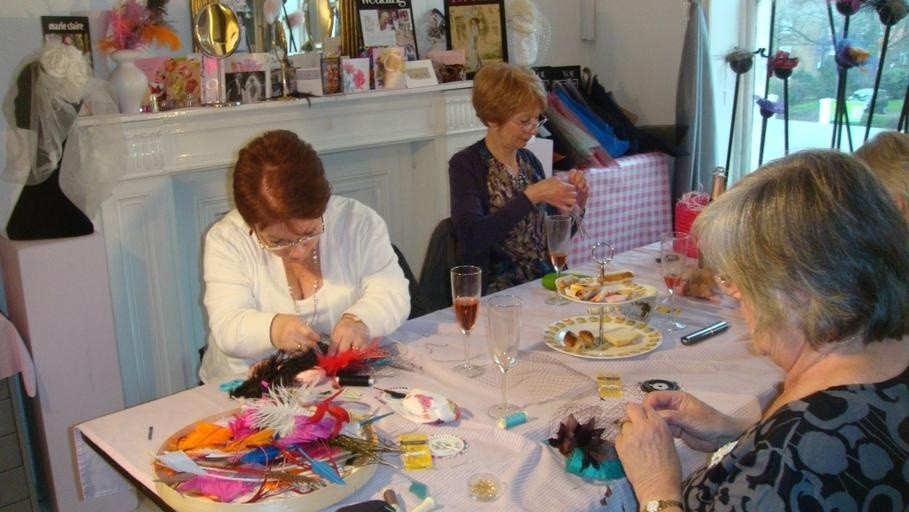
[{"left": 725, "top": 0, "right": 909, "bottom": 184}]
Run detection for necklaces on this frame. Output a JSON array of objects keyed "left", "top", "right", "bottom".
[{"left": 287, "top": 248, "right": 318, "bottom": 326}]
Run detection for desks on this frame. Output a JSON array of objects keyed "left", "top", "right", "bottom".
[{"left": 553, "top": 151, "right": 677, "bottom": 265}]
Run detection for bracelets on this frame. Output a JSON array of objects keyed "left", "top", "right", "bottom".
[{"left": 339, "top": 316, "right": 363, "bottom": 326}]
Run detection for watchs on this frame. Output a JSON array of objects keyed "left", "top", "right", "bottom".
[{"left": 642, "top": 497, "right": 685, "bottom": 512}]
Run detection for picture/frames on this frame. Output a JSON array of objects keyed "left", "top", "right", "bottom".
[
  {"left": 191, "top": 1, "right": 356, "bottom": 65},
  {"left": 356, "top": 1, "right": 419, "bottom": 80},
  {"left": 444, "top": 0, "right": 509, "bottom": 78}
]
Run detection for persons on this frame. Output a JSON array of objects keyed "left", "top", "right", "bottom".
[
  {"left": 448, "top": 62, "right": 591, "bottom": 294},
  {"left": 378, "top": 8, "right": 390, "bottom": 30},
  {"left": 202, "top": 129, "right": 412, "bottom": 388},
  {"left": 469, "top": 18, "right": 483, "bottom": 70},
  {"left": 243, "top": 75, "right": 261, "bottom": 101},
  {"left": 227, "top": 73, "right": 244, "bottom": 102},
  {"left": 852, "top": 131, "right": 909, "bottom": 222},
  {"left": 616, "top": 150, "right": 909, "bottom": 512},
  {"left": 392, "top": 12, "right": 399, "bottom": 28}
]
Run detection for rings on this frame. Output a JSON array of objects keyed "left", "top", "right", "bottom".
[
  {"left": 352, "top": 346, "right": 360, "bottom": 351},
  {"left": 295, "top": 344, "right": 303, "bottom": 351},
  {"left": 619, "top": 417, "right": 632, "bottom": 431}
]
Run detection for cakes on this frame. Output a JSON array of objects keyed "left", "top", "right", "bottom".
[{"left": 555, "top": 270, "right": 634, "bottom": 303}]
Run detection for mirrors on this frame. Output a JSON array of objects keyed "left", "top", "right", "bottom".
[
  {"left": 305, "top": 0, "right": 335, "bottom": 51},
  {"left": 191, "top": 1, "right": 240, "bottom": 59}
]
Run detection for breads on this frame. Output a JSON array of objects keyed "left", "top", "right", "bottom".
[
  {"left": 602, "top": 327, "right": 638, "bottom": 346},
  {"left": 579, "top": 330, "right": 595, "bottom": 348},
  {"left": 563, "top": 331, "right": 583, "bottom": 350}
]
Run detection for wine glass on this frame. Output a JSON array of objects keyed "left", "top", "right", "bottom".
[
  {"left": 451, "top": 266, "right": 482, "bottom": 377},
  {"left": 660, "top": 232, "right": 689, "bottom": 329},
  {"left": 545, "top": 215, "right": 571, "bottom": 305},
  {"left": 488, "top": 295, "right": 521, "bottom": 417}
]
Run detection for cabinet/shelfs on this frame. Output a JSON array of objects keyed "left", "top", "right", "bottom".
[{"left": 76, "top": 81, "right": 488, "bottom": 408}]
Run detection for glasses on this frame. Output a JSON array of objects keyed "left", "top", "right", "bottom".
[
  {"left": 252, "top": 213, "right": 324, "bottom": 252},
  {"left": 505, "top": 109, "right": 548, "bottom": 133}
]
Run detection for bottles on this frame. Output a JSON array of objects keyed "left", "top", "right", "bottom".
[
  {"left": 701, "top": 168, "right": 726, "bottom": 268},
  {"left": 149, "top": 93, "right": 159, "bottom": 113}
]
